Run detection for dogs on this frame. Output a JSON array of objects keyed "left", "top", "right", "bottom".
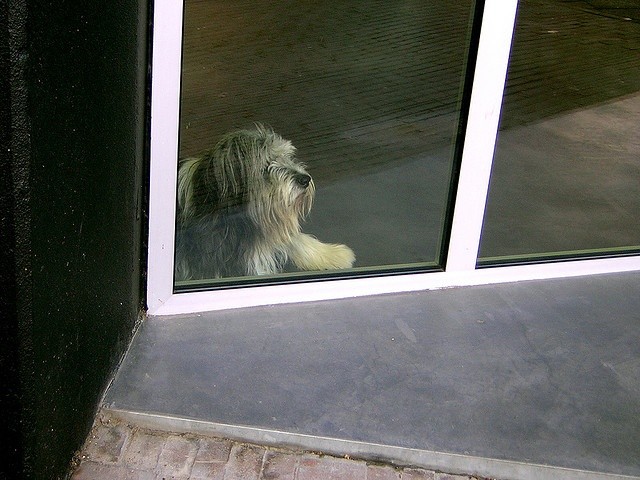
[{"left": 174, "top": 122, "right": 355, "bottom": 280}]
